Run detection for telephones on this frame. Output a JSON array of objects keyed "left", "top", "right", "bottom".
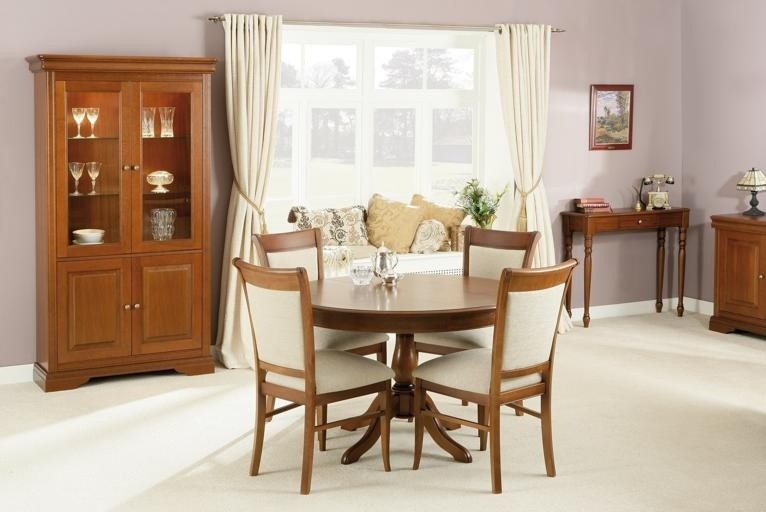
[{"left": 639, "top": 174, "right": 674, "bottom": 211}]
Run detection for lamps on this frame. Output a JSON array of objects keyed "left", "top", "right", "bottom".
[{"left": 734, "top": 167, "right": 766, "bottom": 216}]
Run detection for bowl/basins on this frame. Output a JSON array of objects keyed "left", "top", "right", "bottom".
[{"left": 72, "top": 228, "right": 106, "bottom": 243}]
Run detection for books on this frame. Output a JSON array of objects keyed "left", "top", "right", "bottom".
[{"left": 574, "top": 197, "right": 611, "bottom": 213}]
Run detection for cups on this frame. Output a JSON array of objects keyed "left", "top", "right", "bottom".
[
  {"left": 158, "top": 106, "right": 176, "bottom": 138},
  {"left": 347, "top": 257, "right": 375, "bottom": 287},
  {"left": 141, "top": 106, "right": 156, "bottom": 138},
  {"left": 150, "top": 207, "right": 177, "bottom": 241}
]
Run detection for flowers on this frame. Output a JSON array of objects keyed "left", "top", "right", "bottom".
[{"left": 450, "top": 175, "right": 510, "bottom": 225}]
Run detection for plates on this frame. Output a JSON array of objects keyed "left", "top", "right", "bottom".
[{"left": 71, "top": 240, "right": 105, "bottom": 246}]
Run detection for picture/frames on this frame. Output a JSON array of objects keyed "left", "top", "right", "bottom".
[{"left": 588, "top": 83, "right": 634, "bottom": 150}]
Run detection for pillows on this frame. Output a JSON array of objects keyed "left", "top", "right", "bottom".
[
  {"left": 287, "top": 205, "right": 376, "bottom": 260},
  {"left": 365, "top": 193, "right": 424, "bottom": 254},
  {"left": 408, "top": 195, "right": 465, "bottom": 252},
  {"left": 410, "top": 219, "right": 446, "bottom": 254}
]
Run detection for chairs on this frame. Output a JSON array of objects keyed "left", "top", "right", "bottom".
[
  {"left": 409, "top": 258, "right": 578, "bottom": 492},
  {"left": 232, "top": 257, "right": 397, "bottom": 495},
  {"left": 252, "top": 228, "right": 389, "bottom": 451},
  {"left": 411, "top": 227, "right": 541, "bottom": 406}
]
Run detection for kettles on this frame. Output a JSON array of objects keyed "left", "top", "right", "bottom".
[{"left": 369, "top": 241, "right": 399, "bottom": 280}]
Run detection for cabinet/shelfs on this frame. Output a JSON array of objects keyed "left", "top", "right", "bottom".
[
  {"left": 33, "top": 248, "right": 215, "bottom": 392},
  {"left": 709, "top": 212, "right": 766, "bottom": 336},
  {"left": 25, "top": 54, "right": 217, "bottom": 260}
]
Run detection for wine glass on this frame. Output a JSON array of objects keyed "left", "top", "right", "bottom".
[
  {"left": 84, "top": 162, "right": 101, "bottom": 195},
  {"left": 71, "top": 107, "right": 85, "bottom": 139},
  {"left": 67, "top": 162, "right": 85, "bottom": 197},
  {"left": 85, "top": 107, "right": 100, "bottom": 138}
]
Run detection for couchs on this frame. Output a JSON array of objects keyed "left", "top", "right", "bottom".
[{"left": 294, "top": 207, "right": 473, "bottom": 278}]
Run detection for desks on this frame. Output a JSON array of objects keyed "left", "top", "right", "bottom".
[{"left": 560, "top": 206, "right": 690, "bottom": 328}]
